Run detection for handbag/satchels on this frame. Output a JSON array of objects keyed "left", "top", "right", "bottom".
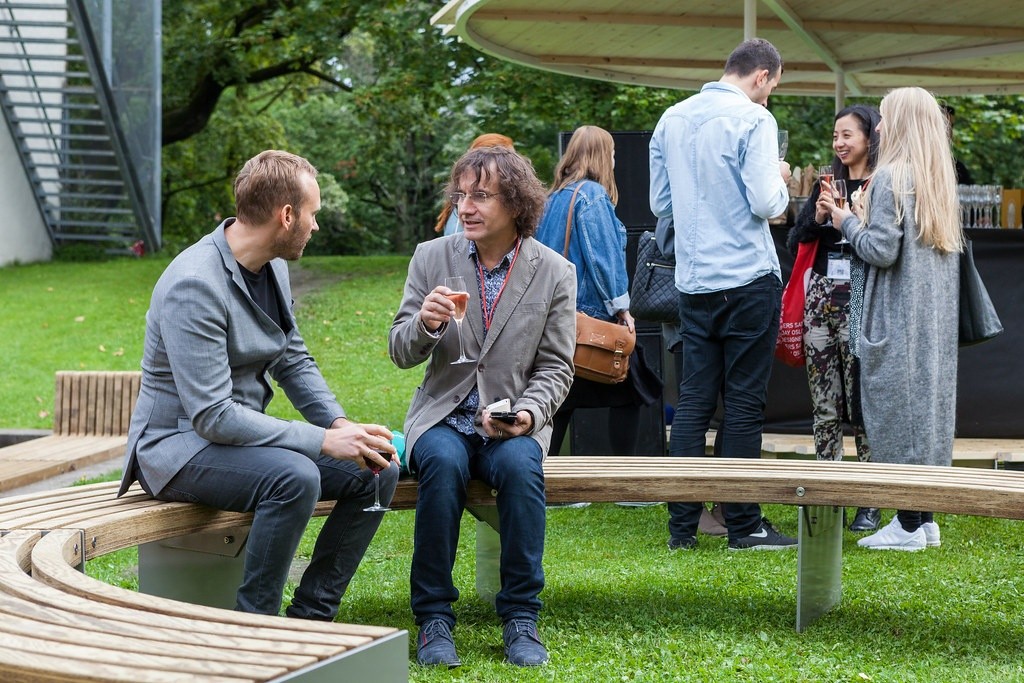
[
  {"left": 629, "top": 230, "right": 682, "bottom": 323},
  {"left": 959, "top": 240, "right": 1003, "bottom": 346},
  {"left": 775, "top": 240, "right": 819, "bottom": 367},
  {"left": 572, "top": 312, "right": 636, "bottom": 383}
]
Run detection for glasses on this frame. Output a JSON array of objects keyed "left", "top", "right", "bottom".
[{"left": 449, "top": 191, "right": 502, "bottom": 205}]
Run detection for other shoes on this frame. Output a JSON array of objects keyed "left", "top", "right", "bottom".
[
  {"left": 699, "top": 503, "right": 729, "bottom": 537},
  {"left": 712, "top": 502, "right": 728, "bottom": 527}
]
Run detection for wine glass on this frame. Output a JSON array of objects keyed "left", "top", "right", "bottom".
[
  {"left": 955, "top": 184, "right": 1005, "bottom": 229},
  {"left": 364, "top": 435, "right": 393, "bottom": 512},
  {"left": 444, "top": 276, "right": 477, "bottom": 365},
  {"left": 818, "top": 165, "right": 837, "bottom": 227},
  {"left": 830, "top": 179, "right": 852, "bottom": 244}
]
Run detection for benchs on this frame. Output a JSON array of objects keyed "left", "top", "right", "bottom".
[
  {"left": 0, "top": 455, "right": 1024, "bottom": 683},
  {"left": 0, "top": 370, "right": 152, "bottom": 491}
]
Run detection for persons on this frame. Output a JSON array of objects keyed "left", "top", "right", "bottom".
[
  {"left": 532, "top": 125, "right": 664, "bottom": 507},
  {"left": 827, "top": 86, "right": 970, "bottom": 551},
  {"left": 117, "top": 149, "right": 401, "bottom": 622},
  {"left": 436, "top": 133, "right": 517, "bottom": 235},
  {"left": 649, "top": 38, "right": 797, "bottom": 551},
  {"left": 939, "top": 105, "right": 992, "bottom": 227},
  {"left": 794, "top": 102, "right": 883, "bottom": 531},
  {"left": 390, "top": 145, "right": 577, "bottom": 667}
]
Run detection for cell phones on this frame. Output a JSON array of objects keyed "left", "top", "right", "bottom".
[{"left": 489, "top": 411, "right": 518, "bottom": 425}]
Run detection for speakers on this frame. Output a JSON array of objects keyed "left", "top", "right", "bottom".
[{"left": 559, "top": 130, "right": 668, "bottom": 456}]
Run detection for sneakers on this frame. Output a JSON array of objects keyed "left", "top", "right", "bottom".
[
  {"left": 922, "top": 520, "right": 941, "bottom": 547},
  {"left": 502, "top": 617, "right": 548, "bottom": 667},
  {"left": 416, "top": 617, "right": 461, "bottom": 668},
  {"left": 727, "top": 516, "right": 798, "bottom": 552},
  {"left": 667, "top": 535, "right": 694, "bottom": 552},
  {"left": 856, "top": 514, "right": 927, "bottom": 553}
]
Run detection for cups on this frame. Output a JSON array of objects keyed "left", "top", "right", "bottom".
[{"left": 777, "top": 129, "right": 789, "bottom": 162}]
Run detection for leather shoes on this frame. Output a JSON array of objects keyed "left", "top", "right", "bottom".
[{"left": 849, "top": 508, "right": 882, "bottom": 532}]
